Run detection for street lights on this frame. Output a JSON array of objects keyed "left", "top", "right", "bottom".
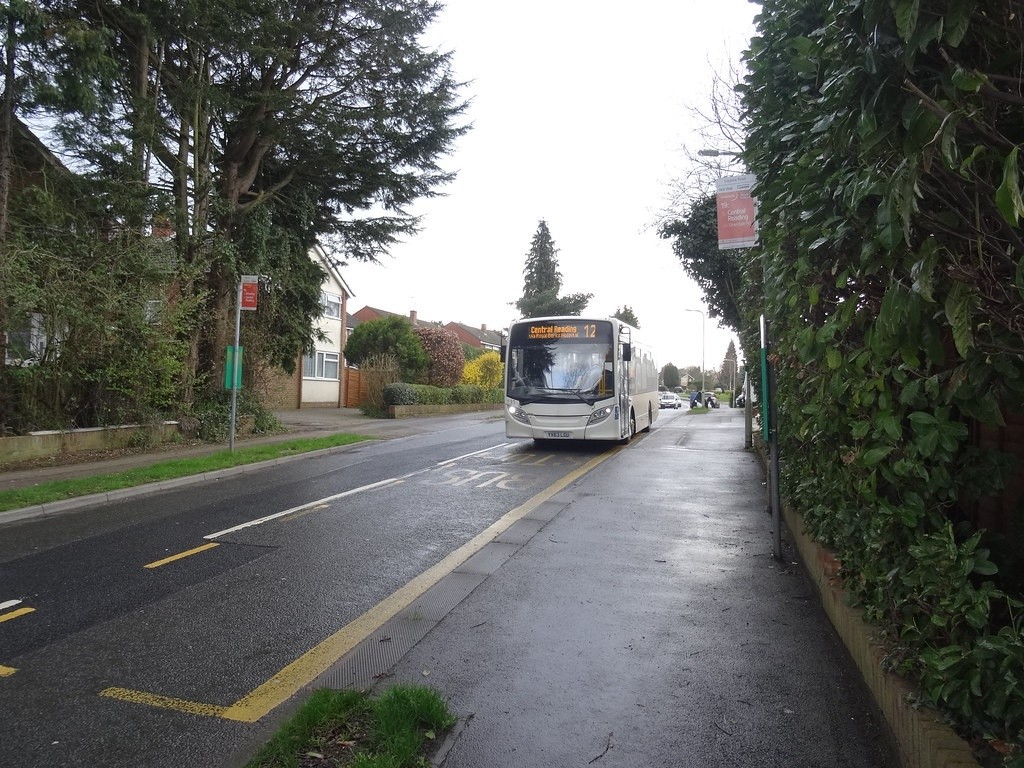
[
  {"left": 685, "top": 308, "right": 705, "bottom": 408},
  {"left": 725, "top": 359, "right": 735, "bottom": 408}
]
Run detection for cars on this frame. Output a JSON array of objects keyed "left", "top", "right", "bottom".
[
  {"left": 688, "top": 390, "right": 698, "bottom": 407},
  {"left": 659, "top": 385, "right": 669, "bottom": 391},
  {"left": 21, "top": 357, "right": 41, "bottom": 367},
  {"left": 658, "top": 392, "right": 663, "bottom": 409},
  {"left": 704, "top": 391, "right": 720, "bottom": 408},
  {"left": 736, "top": 393, "right": 745, "bottom": 408},
  {"left": 677, "top": 394, "right": 682, "bottom": 407}
]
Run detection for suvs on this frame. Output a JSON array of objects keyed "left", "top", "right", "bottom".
[
  {"left": 660, "top": 394, "right": 678, "bottom": 409},
  {"left": 673, "top": 386, "right": 683, "bottom": 393}
]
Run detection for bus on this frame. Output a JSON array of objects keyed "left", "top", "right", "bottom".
[{"left": 500, "top": 315, "right": 659, "bottom": 444}]
